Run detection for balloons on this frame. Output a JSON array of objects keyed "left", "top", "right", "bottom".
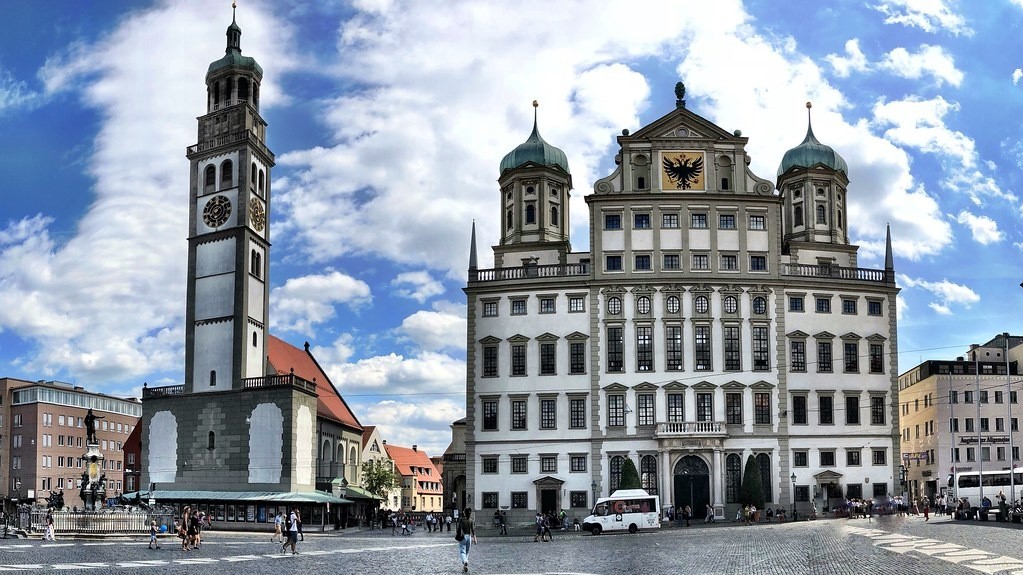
[{"left": 161, "top": 525, "right": 167, "bottom": 532}]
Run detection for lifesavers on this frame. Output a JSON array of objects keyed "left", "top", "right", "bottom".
[{"left": 614, "top": 501, "right": 626, "bottom": 513}]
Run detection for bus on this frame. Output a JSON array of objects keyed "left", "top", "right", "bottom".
[{"left": 955, "top": 466, "right": 1023, "bottom": 509}]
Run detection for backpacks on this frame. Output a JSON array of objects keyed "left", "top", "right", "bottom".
[{"left": 286, "top": 513, "right": 295, "bottom": 529}]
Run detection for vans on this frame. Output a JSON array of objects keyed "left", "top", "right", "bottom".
[{"left": 582, "top": 489, "right": 661, "bottom": 535}]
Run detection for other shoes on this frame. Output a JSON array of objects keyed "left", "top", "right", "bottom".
[{"left": 464, "top": 563, "right": 468, "bottom": 572}]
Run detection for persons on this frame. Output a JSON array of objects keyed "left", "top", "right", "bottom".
[
  {"left": 846, "top": 498, "right": 919, "bottom": 519},
  {"left": 704, "top": 504, "right": 716, "bottom": 525},
  {"left": 270, "top": 505, "right": 304, "bottom": 554},
  {"left": 46, "top": 508, "right": 57, "bottom": 542},
  {"left": 174, "top": 506, "right": 212, "bottom": 550},
  {"left": 735, "top": 503, "right": 788, "bottom": 523},
  {"left": 919, "top": 490, "right": 1007, "bottom": 523},
  {"left": 533, "top": 509, "right": 581, "bottom": 542},
  {"left": 84, "top": 408, "right": 105, "bottom": 444},
  {"left": 668, "top": 505, "right": 692, "bottom": 528},
  {"left": 806, "top": 498, "right": 818, "bottom": 521},
  {"left": 392, "top": 506, "right": 477, "bottom": 572},
  {"left": 494, "top": 509, "right": 507, "bottom": 535},
  {"left": 148, "top": 520, "right": 162, "bottom": 549}
]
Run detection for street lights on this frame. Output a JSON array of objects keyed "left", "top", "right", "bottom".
[
  {"left": 905, "top": 468, "right": 909, "bottom": 492},
  {"left": 790, "top": 473, "right": 797, "bottom": 523}
]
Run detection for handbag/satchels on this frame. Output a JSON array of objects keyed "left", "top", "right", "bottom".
[{"left": 455, "top": 518, "right": 465, "bottom": 541}]
